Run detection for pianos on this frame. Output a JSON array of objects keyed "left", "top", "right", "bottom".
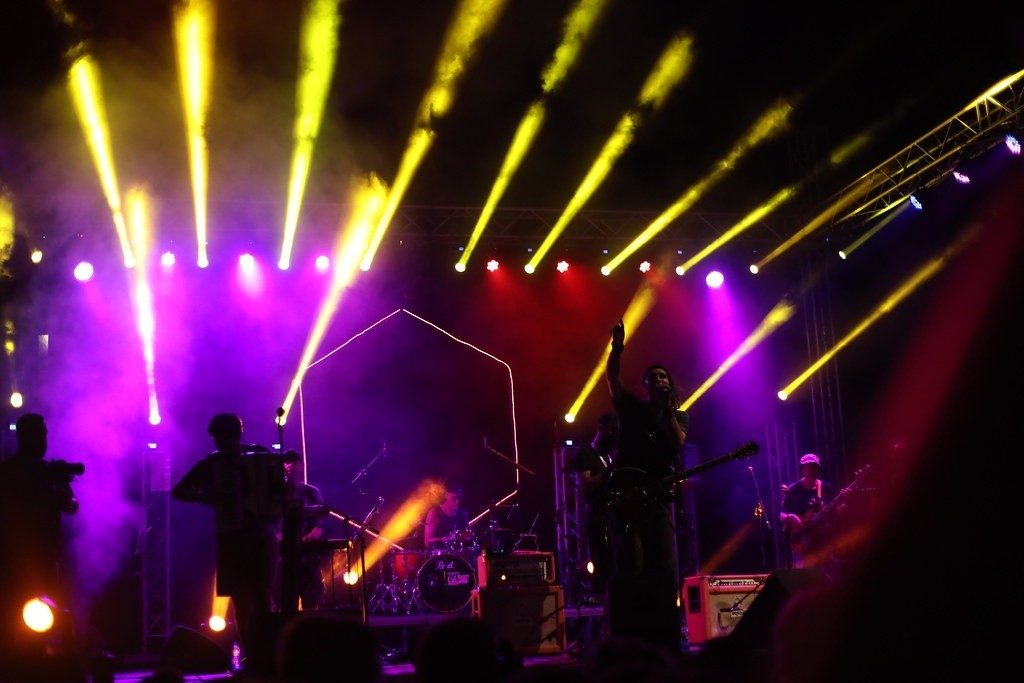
[{"left": 276, "top": 536, "right": 354, "bottom": 613}]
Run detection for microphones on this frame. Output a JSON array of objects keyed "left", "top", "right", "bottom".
[
  {"left": 747, "top": 466, "right": 753, "bottom": 472},
  {"left": 483, "top": 436, "right": 486, "bottom": 449},
  {"left": 719, "top": 608, "right": 742, "bottom": 612},
  {"left": 277, "top": 406, "right": 285, "bottom": 416},
  {"left": 662, "top": 388, "right": 669, "bottom": 393},
  {"left": 383, "top": 443, "right": 391, "bottom": 465}
]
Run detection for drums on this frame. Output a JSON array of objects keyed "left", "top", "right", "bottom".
[
  {"left": 417, "top": 552, "right": 477, "bottom": 612},
  {"left": 393, "top": 549, "right": 425, "bottom": 593},
  {"left": 429, "top": 535, "right": 478, "bottom": 570}
]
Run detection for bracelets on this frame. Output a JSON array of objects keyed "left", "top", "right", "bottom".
[{"left": 612, "top": 341, "right": 625, "bottom": 353}]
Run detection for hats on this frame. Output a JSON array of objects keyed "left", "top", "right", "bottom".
[
  {"left": 282, "top": 447, "right": 302, "bottom": 464},
  {"left": 800, "top": 453, "right": 820, "bottom": 466},
  {"left": 446, "top": 478, "right": 464, "bottom": 493}
]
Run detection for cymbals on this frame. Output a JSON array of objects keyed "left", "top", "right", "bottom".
[{"left": 488, "top": 528, "right": 514, "bottom": 531}]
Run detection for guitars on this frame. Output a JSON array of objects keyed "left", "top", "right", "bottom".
[
  {"left": 607, "top": 440, "right": 760, "bottom": 525},
  {"left": 782, "top": 463, "right": 873, "bottom": 553}
]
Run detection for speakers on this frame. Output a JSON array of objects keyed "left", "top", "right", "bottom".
[
  {"left": 156, "top": 625, "right": 231, "bottom": 675},
  {"left": 472, "top": 586, "right": 566, "bottom": 654},
  {"left": 684, "top": 573, "right": 767, "bottom": 644}
]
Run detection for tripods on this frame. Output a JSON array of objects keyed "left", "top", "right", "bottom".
[{"left": 369, "top": 543, "right": 423, "bottom": 616}]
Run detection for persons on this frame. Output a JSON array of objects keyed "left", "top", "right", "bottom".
[
  {"left": 607, "top": 318, "right": 691, "bottom": 651},
  {"left": 422, "top": 479, "right": 469, "bottom": 557},
  {"left": 171, "top": 412, "right": 284, "bottom": 667},
  {"left": 279, "top": 450, "right": 325, "bottom": 613},
  {"left": 570, "top": 412, "right": 618, "bottom": 608},
  {"left": 779, "top": 452, "right": 837, "bottom": 536},
  {"left": 1, "top": 413, "right": 79, "bottom": 583}
]
